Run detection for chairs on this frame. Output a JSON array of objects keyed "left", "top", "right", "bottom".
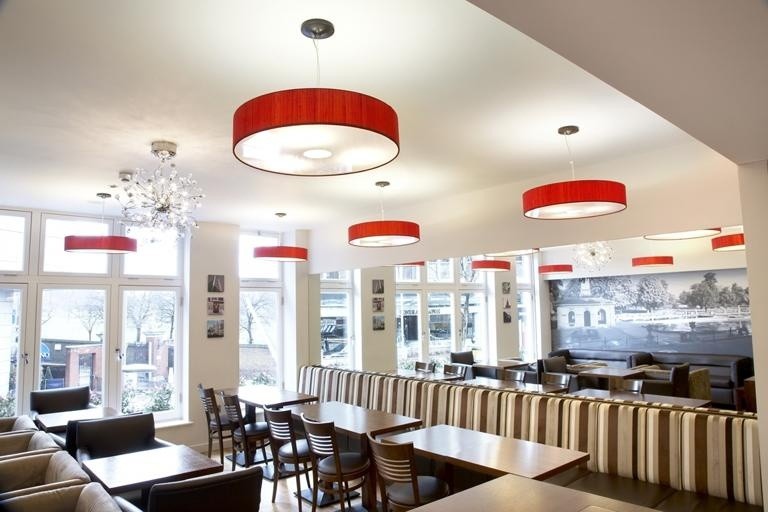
[
  {"left": 197, "top": 379, "right": 449, "bottom": 511},
  {"left": 0, "top": 387, "right": 263, "bottom": 512}
]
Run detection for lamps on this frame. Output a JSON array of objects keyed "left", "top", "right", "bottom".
[
  {"left": 471, "top": 260, "right": 510, "bottom": 271},
  {"left": 384, "top": 262, "right": 425, "bottom": 268},
  {"left": 64, "top": 197, "right": 137, "bottom": 254},
  {"left": 129, "top": 140, "right": 207, "bottom": 244},
  {"left": 348, "top": 192, "right": 420, "bottom": 247},
  {"left": 253, "top": 219, "right": 309, "bottom": 262},
  {"left": 632, "top": 256, "right": 673, "bottom": 266},
  {"left": 539, "top": 265, "right": 573, "bottom": 274},
  {"left": 109, "top": 169, "right": 145, "bottom": 228},
  {"left": 712, "top": 234, "right": 745, "bottom": 252},
  {"left": 232, "top": 35, "right": 400, "bottom": 177},
  {"left": 521, "top": 132, "right": 627, "bottom": 221},
  {"left": 642, "top": 227, "right": 722, "bottom": 242}
]
